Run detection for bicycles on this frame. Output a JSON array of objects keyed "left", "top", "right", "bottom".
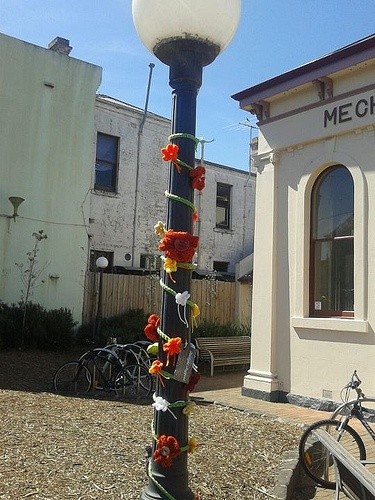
[
  {"left": 298, "top": 368, "right": 375, "bottom": 489},
  {"left": 52, "top": 332, "right": 160, "bottom": 403}
]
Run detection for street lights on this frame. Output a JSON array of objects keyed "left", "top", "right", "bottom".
[
  {"left": 125, "top": 0, "right": 242, "bottom": 500},
  {"left": 95, "top": 257, "right": 108, "bottom": 331}
]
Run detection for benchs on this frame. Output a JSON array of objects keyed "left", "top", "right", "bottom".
[{"left": 191, "top": 336, "right": 251, "bottom": 377}]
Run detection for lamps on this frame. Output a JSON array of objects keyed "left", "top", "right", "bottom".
[{"left": 9, "top": 197, "right": 25, "bottom": 223}]
